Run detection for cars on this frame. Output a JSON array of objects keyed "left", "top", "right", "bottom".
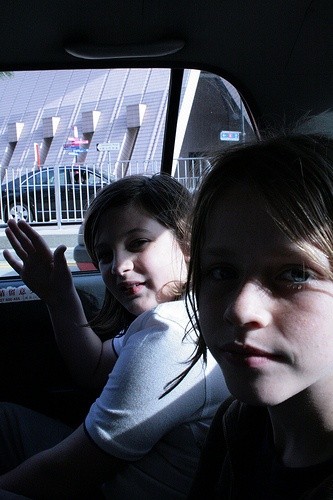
[{"left": 0, "top": 165, "right": 118, "bottom": 226}]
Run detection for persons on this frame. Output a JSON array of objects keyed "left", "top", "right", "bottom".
[
  {"left": 74, "top": 174, "right": 85, "bottom": 184},
  {"left": 160, "top": 133, "right": 333, "bottom": 500},
  {"left": 0, "top": 173, "right": 232, "bottom": 500}
]
